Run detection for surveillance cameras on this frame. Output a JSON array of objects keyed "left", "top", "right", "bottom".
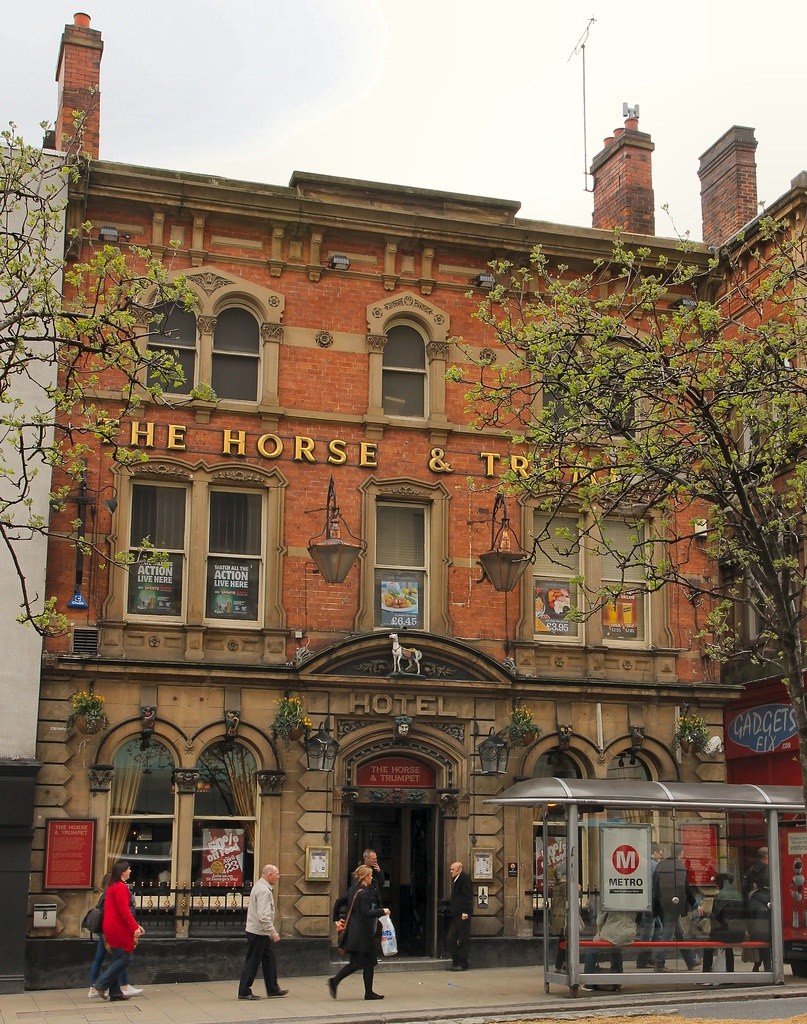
[{"left": 703, "top": 736, "right": 721, "bottom": 754}]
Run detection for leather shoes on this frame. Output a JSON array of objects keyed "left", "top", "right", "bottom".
[
  {"left": 268, "top": 990, "right": 288, "bottom": 996},
  {"left": 238, "top": 995, "right": 261, "bottom": 1000}
]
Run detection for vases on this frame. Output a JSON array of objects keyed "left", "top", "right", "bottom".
[
  {"left": 285, "top": 725, "right": 305, "bottom": 742},
  {"left": 509, "top": 732, "right": 536, "bottom": 747},
  {"left": 74, "top": 716, "right": 103, "bottom": 735},
  {"left": 679, "top": 737, "right": 701, "bottom": 754}
]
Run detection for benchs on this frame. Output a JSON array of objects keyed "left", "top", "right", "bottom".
[{"left": 559, "top": 940, "right": 771, "bottom": 951}]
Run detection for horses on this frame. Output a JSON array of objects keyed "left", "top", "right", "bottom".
[{"left": 389, "top": 633, "right": 422, "bottom": 675}]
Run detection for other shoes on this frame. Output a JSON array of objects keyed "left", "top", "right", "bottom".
[
  {"left": 327, "top": 977, "right": 337, "bottom": 1000},
  {"left": 690, "top": 960, "right": 702, "bottom": 969},
  {"left": 696, "top": 982, "right": 711, "bottom": 986},
  {"left": 110, "top": 993, "right": 131, "bottom": 1000},
  {"left": 609, "top": 983, "right": 623, "bottom": 994},
  {"left": 365, "top": 992, "right": 385, "bottom": 1000},
  {"left": 88, "top": 990, "right": 99, "bottom": 998},
  {"left": 654, "top": 965, "right": 672, "bottom": 973},
  {"left": 582, "top": 984, "right": 595, "bottom": 992},
  {"left": 446, "top": 965, "right": 468, "bottom": 972},
  {"left": 123, "top": 985, "right": 143, "bottom": 996},
  {"left": 93, "top": 982, "right": 107, "bottom": 1000},
  {"left": 554, "top": 967, "right": 566, "bottom": 974}
]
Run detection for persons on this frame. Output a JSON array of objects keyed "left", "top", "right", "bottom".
[
  {"left": 698, "top": 857, "right": 720, "bottom": 883},
  {"left": 579, "top": 901, "right": 639, "bottom": 992},
  {"left": 684, "top": 859, "right": 697, "bottom": 884},
  {"left": 552, "top": 881, "right": 582, "bottom": 973},
  {"left": 350, "top": 848, "right": 385, "bottom": 962},
  {"left": 439, "top": 861, "right": 474, "bottom": 971},
  {"left": 636, "top": 842, "right": 664, "bottom": 970},
  {"left": 651, "top": 842, "right": 702, "bottom": 973},
  {"left": 702, "top": 873, "right": 742, "bottom": 972},
  {"left": 237, "top": 864, "right": 290, "bottom": 1000},
  {"left": 87, "top": 873, "right": 144, "bottom": 997},
  {"left": 91, "top": 859, "right": 145, "bottom": 1002},
  {"left": 742, "top": 847, "right": 774, "bottom": 972},
  {"left": 328, "top": 863, "right": 387, "bottom": 1001}
]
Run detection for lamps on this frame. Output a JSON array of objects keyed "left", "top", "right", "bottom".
[
  {"left": 98, "top": 226, "right": 120, "bottom": 243},
  {"left": 467, "top": 273, "right": 496, "bottom": 289},
  {"left": 320, "top": 255, "right": 351, "bottom": 271},
  {"left": 305, "top": 721, "right": 339, "bottom": 773},
  {"left": 305, "top": 475, "right": 368, "bottom": 584},
  {"left": 49, "top": 483, "right": 118, "bottom": 514},
  {"left": 466, "top": 493, "right": 536, "bottom": 593},
  {"left": 476, "top": 726, "right": 509, "bottom": 774},
  {"left": 668, "top": 299, "right": 698, "bottom": 311}
]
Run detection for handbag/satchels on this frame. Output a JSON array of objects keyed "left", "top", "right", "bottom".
[
  {"left": 562, "top": 912, "right": 586, "bottom": 939},
  {"left": 741, "top": 931, "right": 760, "bottom": 962},
  {"left": 710, "top": 948, "right": 728, "bottom": 987},
  {"left": 335, "top": 925, "right": 346, "bottom": 955},
  {"left": 85, "top": 908, "right": 102, "bottom": 933},
  {"left": 378, "top": 915, "right": 398, "bottom": 957}
]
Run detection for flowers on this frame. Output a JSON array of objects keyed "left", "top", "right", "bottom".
[
  {"left": 667, "top": 713, "right": 711, "bottom": 756},
  {"left": 269, "top": 696, "right": 313, "bottom": 749},
  {"left": 64, "top": 691, "right": 110, "bottom": 733},
  {"left": 498, "top": 704, "right": 544, "bottom": 748}
]
